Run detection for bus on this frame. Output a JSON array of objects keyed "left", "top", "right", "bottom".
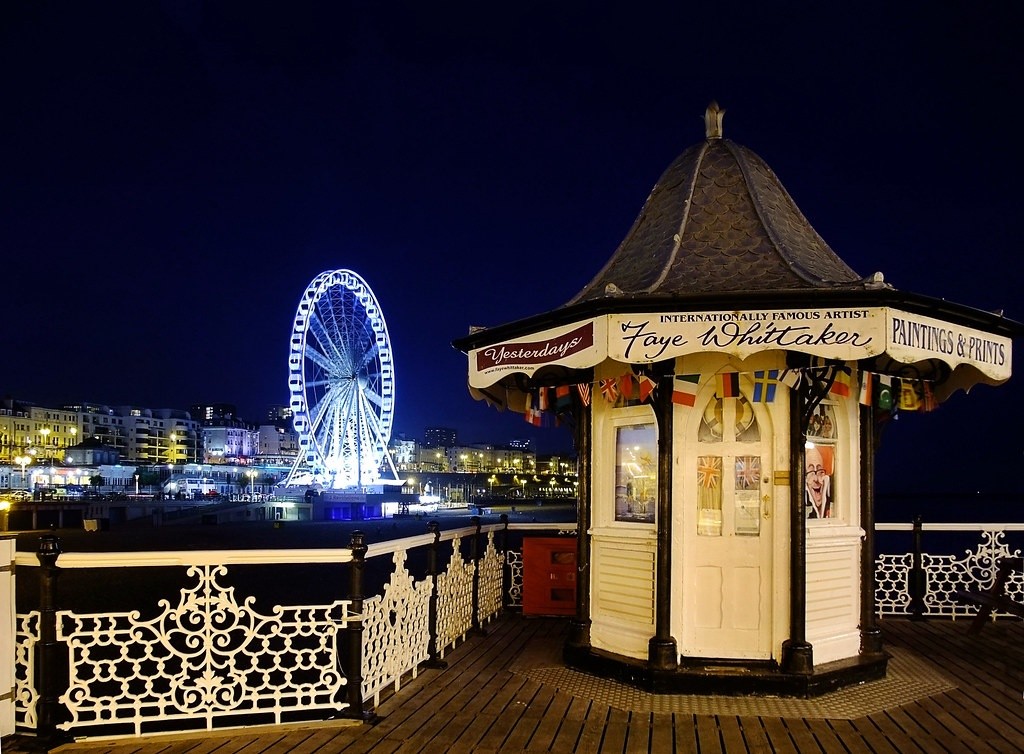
[{"left": 177, "top": 478, "right": 216, "bottom": 499}]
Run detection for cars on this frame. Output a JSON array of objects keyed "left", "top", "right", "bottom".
[
  {"left": 0, "top": 490, "right": 33, "bottom": 503},
  {"left": 79, "top": 492, "right": 105, "bottom": 502},
  {"left": 241, "top": 491, "right": 275, "bottom": 503},
  {"left": 155, "top": 492, "right": 176, "bottom": 500},
  {"left": 102, "top": 491, "right": 131, "bottom": 501}
]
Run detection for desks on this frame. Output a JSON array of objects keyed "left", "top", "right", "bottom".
[{"left": 967, "top": 558, "right": 1024, "bottom": 636}]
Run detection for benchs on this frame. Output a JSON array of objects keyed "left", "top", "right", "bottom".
[{"left": 959, "top": 589, "right": 1024, "bottom": 618}]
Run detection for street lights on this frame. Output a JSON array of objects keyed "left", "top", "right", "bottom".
[
  {"left": 246, "top": 469, "right": 257, "bottom": 502},
  {"left": 71, "top": 428, "right": 78, "bottom": 445},
  {"left": 170, "top": 432, "right": 177, "bottom": 464},
  {"left": 16, "top": 457, "right": 31, "bottom": 503},
  {"left": 38, "top": 429, "right": 50, "bottom": 450}
]
